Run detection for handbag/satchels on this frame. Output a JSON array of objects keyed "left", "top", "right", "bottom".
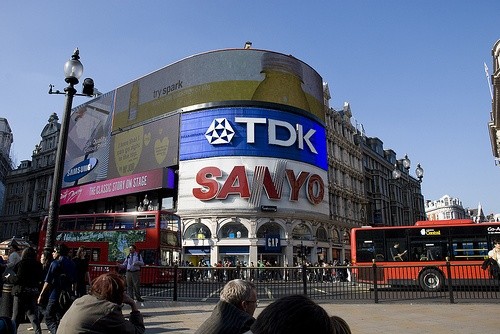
[{"left": 59, "top": 287, "right": 71, "bottom": 310}]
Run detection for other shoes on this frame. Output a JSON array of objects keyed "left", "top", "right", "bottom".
[
  {"left": 35, "top": 328, "right": 41, "bottom": 334},
  {"left": 138, "top": 299, "right": 144, "bottom": 302}
]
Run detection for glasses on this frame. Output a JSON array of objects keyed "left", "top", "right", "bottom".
[{"left": 241, "top": 299, "right": 260, "bottom": 307}]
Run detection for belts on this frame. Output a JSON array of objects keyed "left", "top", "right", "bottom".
[{"left": 128, "top": 271, "right": 138, "bottom": 272}]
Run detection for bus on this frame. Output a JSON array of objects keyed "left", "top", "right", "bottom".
[
  {"left": 350, "top": 219, "right": 500, "bottom": 292},
  {"left": 37, "top": 209, "right": 181, "bottom": 288}
]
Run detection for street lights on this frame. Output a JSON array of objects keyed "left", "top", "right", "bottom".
[
  {"left": 137, "top": 194, "right": 153, "bottom": 211},
  {"left": 392, "top": 153, "right": 424, "bottom": 225},
  {"left": 42, "top": 47, "right": 95, "bottom": 271}
]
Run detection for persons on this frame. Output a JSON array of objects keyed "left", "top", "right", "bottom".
[
  {"left": 116, "top": 245, "right": 145, "bottom": 302},
  {"left": 193, "top": 279, "right": 260, "bottom": 334},
  {"left": 243, "top": 294, "right": 351, "bottom": 334},
  {"left": 0, "top": 243, "right": 22, "bottom": 321},
  {"left": 184, "top": 259, "right": 351, "bottom": 283},
  {"left": 392, "top": 243, "right": 408, "bottom": 261},
  {"left": 56, "top": 272, "right": 146, "bottom": 334},
  {"left": 481, "top": 239, "right": 500, "bottom": 280},
  {"left": 70, "top": 246, "right": 92, "bottom": 298},
  {"left": 37, "top": 243, "right": 77, "bottom": 334},
  {"left": 5, "top": 247, "right": 42, "bottom": 334},
  {"left": 28, "top": 252, "right": 51, "bottom": 334}
]
